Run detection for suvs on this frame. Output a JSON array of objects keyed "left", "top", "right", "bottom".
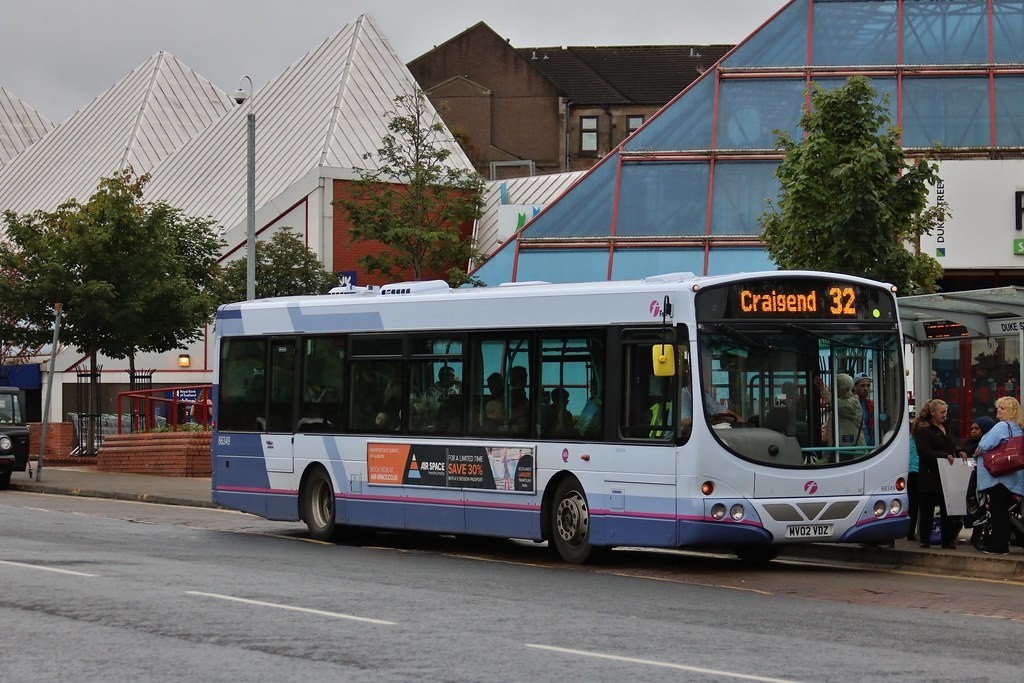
[{"left": 0, "top": 386, "right": 30, "bottom": 490}]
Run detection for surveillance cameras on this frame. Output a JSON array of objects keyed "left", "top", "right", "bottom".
[{"left": 234, "top": 90, "right": 246, "bottom": 104}]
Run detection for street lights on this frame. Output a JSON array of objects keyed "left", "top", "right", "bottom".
[{"left": 232, "top": 74, "right": 256, "bottom": 301}]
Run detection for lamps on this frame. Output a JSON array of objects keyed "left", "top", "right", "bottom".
[{"left": 179, "top": 354, "right": 190, "bottom": 367}]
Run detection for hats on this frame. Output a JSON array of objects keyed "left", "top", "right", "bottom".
[{"left": 853, "top": 373, "right": 870, "bottom": 384}]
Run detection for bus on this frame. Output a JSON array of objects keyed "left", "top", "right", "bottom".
[{"left": 210, "top": 269, "right": 911, "bottom": 564}]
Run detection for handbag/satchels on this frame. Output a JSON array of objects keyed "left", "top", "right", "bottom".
[{"left": 981, "top": 421, "right": 1024, "bottom": 477}]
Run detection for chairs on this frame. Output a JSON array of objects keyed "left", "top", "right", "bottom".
[{"left": 648, "top": 401, "right": 671, "bottom": 439}]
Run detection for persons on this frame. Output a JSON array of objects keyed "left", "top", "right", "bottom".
[
  {"left": 781, "top": 381, "right": 808, "bottom": 434},
  {"left": 486, "top": 365, "right": 582, "bottom": 440},
  {"left": 814, "top": 374, "right": 868, "bottom": 463},
  {"left": 665, "top": 373, "right": 744, "bottom": 438},
  {"left": 354, "top": 365, "right": 402, "bottom": 435},
  {"left": 907, "top": 398, "right": 967, "bottom": 549},
  {"left": 932, "top": 364, "right": 1016, "bottom": 412},
  {"left": 851, "top": 373, "right": 876, "bottom": 446},
  {"left": 428, "top": 365, "right": 462, "bottom": 400},
  {"left": 173, "top": 396, "right": 186, "bottom": 424},
  {"left": 190, "top": 390, "right": 213, "bottom": 424},
  {"left": 962, "top": 397, "right": 1024, "bottom": 555}
]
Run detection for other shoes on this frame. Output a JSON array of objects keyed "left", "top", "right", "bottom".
[
  {"left": 919, "top": 539, "right": 929, "bottom": 547},
  {"left": 877, "top": 539, "right": 894, "bottom": 549},
  {"left": 942, "top": 542, "right": 956, "bottom": 549},
  {"left": 983, "top": 546, "right": 1010, "bottom": 556},
  {"left": 908, "top": 534, "right": 916, "bottom": 540}
]
